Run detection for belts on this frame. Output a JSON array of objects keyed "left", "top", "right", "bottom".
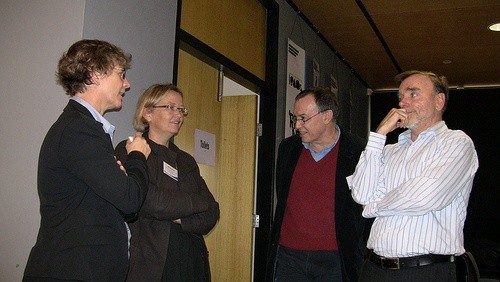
[{"left": 366, "top": 249, "right": 451, "bottom": 271}]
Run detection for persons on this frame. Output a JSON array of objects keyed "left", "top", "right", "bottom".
[
  {"left": 114, "top": 83, "right": 221, "bottom": 282},
  {"left": 345, "top": 70, "right": 478, "bottom": 282},
  {"left": 269, "top": 87, "right": 365, "bottom": 282},
  {"left": 21, "top": 39, "right": 152, "bottom": 282}
]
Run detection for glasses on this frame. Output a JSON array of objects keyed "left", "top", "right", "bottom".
[
  {"left": 291, "top": 108, "right": 330, "bottom": 125},
  {"left": 151, "top": 105, "right": 188, "bottom": 117}
]
